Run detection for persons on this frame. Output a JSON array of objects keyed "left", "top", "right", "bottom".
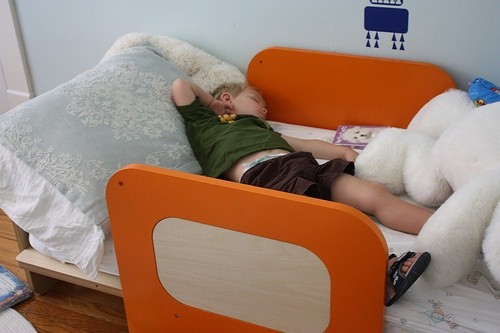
[{"left": 171, "top": 76, "right": 435, "bottom": 306}]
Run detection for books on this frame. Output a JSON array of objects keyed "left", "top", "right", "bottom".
[{"left": 0, "top": 265, "right": 33, "bottom": 313}]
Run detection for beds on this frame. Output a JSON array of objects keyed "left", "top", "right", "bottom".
[{"left": 10, "top": 46, "right": 500, "bottom": 333}]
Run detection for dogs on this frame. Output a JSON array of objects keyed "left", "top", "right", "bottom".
[{"left": 339, "top": 124, "right": 381, "bottom": 143}]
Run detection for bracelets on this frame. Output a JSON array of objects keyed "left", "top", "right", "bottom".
[{"left": 207, "top": 97, "right": 214, "bottom": 108}]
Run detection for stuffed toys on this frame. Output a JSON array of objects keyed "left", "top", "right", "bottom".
[{"left": 353, "top": 89, "right": 500, "bottom": 289}]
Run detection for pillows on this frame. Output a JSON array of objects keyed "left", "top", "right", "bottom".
[{"left": 0, "top": 46, "right": 203, "bottom": 279}]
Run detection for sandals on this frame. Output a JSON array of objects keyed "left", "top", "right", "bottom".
[{"left": 383, "top": 251, "right": 431, "bottom": 308}]
